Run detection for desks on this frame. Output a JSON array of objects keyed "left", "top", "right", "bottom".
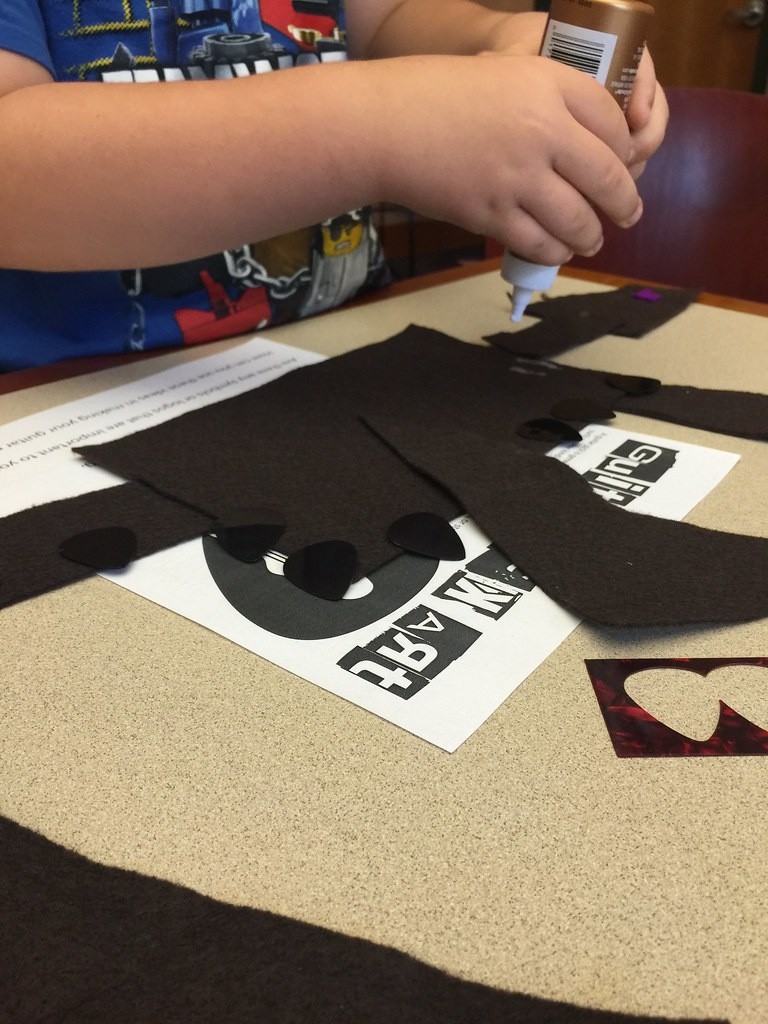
[{"left": 0, "top": 246, "right": 768, "bottom": 1024}]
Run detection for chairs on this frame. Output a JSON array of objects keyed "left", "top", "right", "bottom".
[{"left": 564, "top": 85, "right": 768, "bottom": 305}]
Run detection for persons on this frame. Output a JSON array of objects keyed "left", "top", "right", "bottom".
[{"left": 1, "top": 0, "right": 670, "bottom": 371}]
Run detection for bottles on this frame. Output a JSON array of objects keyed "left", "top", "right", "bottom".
[{"left": 500, "top": 0, "right": 655, "bottom": 323}]
